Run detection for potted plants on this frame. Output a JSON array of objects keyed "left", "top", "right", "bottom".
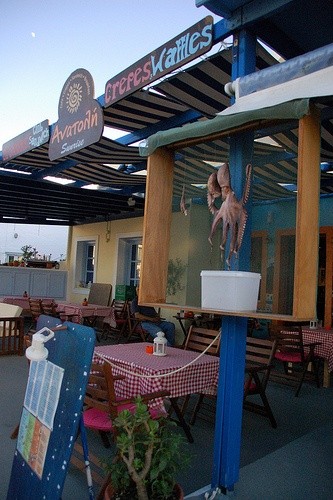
[{"left": 99, "top": 392, "right": 197, "bottom": 500}]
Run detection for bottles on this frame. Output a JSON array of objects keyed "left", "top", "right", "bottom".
[
  {"left": 188, "top": 311, "right": 193, "bottom": 318},
  {"left": 76, "top": 282, "right": 81, "bottom": 288},
  {"left": 180, "top": 310, "right": 184, "bottom": 317},
  {"left": 88, "top": 281, "right": 91, "bottom": 288}
]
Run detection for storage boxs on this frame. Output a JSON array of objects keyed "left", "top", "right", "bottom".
[
  {"left": 115, "top": 285, "right": 136, "bottom": 301},
  {"left": 200, "top": 270, "right": 261, "bottom": 313}
]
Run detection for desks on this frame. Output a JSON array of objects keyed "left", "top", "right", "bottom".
[
  {"left": 4, "top": 298, "right": 117, "bottom": 343},
  {"left": 280, "top": 328, "right": 333, "bottom": 388},
  {"left": 90, "top": 343, "right": 220, "bottom": 444},
  {"left": 173, "top": 316, "right": 221, "bottom": 337}
]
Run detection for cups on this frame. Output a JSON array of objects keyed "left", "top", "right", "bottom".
[
  {"left": 325, "top": 323, "right": 330, "bottom": 332},
  {"left": 316, "top": 320, "right": 322, "bottom": 332}
]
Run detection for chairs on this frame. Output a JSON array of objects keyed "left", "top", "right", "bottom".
[
  {"left": 0, "top": 297, "right": 166, "bottom": 356},
  {"left": 83, "top": 325, "right": 323, "bottom": 448}
]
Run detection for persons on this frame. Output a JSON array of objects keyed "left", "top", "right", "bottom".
[{"left": 132, "top": 285, "right": 176, "bottom": 347}]
